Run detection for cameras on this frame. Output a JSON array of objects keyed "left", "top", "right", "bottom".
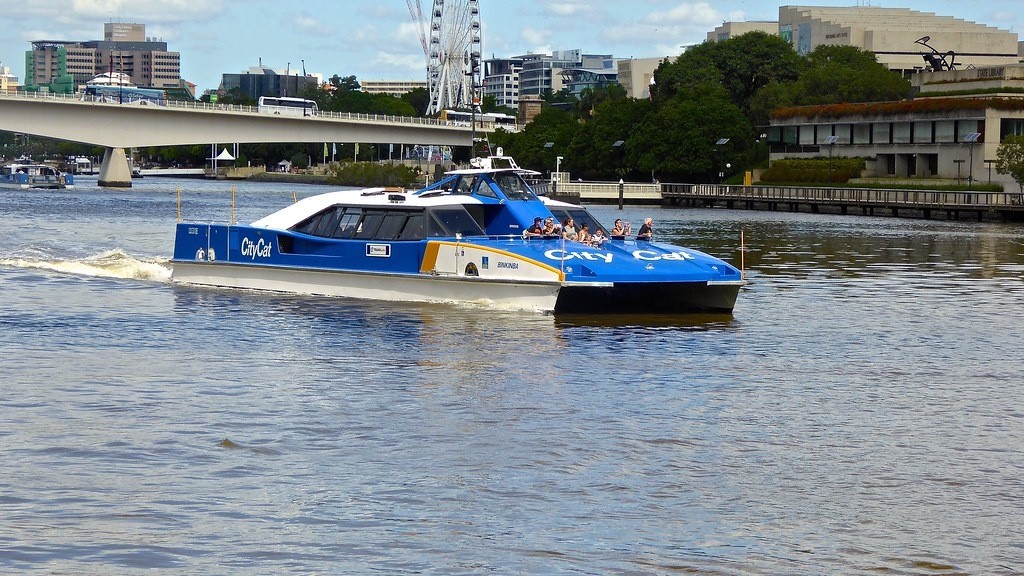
[{"left": 624, "top": 222, "right": 629, "bottom": 227}]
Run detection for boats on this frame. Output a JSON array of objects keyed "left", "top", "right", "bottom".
[
  {"left": 169, "top": 170, "right": 749, "bottom": 315},
  {"left": 0, "top": 163, "right": 74, "bottom": 192}
]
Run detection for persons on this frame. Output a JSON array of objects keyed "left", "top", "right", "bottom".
[
  {"left": 527, "top": 217, "right": 603, "bottom": 245},
  {"left": 611, "top": 219, "right": 631, "bottom": 240},
  {"left": 637, "top": 218, "right": 654, "bottom": 240}
]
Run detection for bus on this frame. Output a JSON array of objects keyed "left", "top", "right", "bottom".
[
  {"left": 440, "top": 110, "right": 516, "bottom": 132},
  {"left": 83, "top": 86, "right": 166, "bottom": 107},
  {"left": 258, "top": 95, "right": 318, "bottom": 117}
]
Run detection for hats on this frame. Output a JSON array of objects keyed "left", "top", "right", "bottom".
[{"left": 534, "top": 217, "right": 543, "bottom": 223}]
